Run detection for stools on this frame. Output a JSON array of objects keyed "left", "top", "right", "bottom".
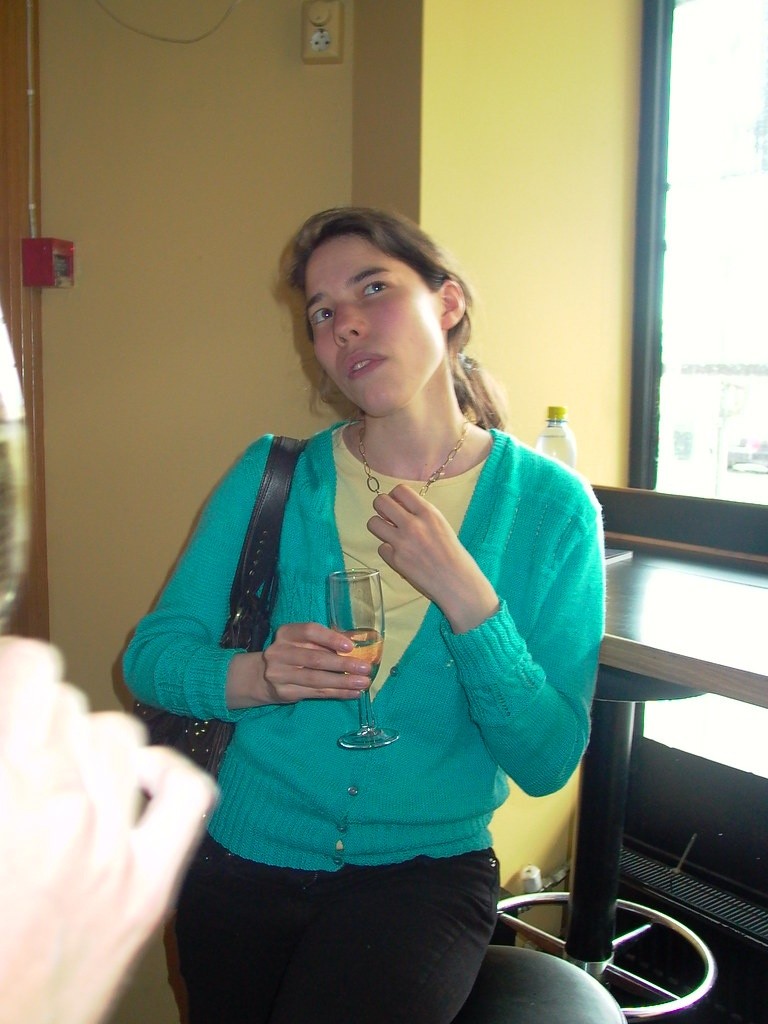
[
  {"left": 453, "top": 944, "right": 628, "bottom": 1024},
  {"left": 494, "top": 665, "right": 720, "bottom": 1024}
]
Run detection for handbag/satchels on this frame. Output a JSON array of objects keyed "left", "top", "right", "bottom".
[{"left": 132, "top": 435, "right": 311, "bottom": 780}]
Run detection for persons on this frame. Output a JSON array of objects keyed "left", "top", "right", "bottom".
[{"left": 119, "top": 206, "right": 606, "bottom": 1023}]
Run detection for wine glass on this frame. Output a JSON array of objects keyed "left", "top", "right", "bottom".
[{"left": 329, "top": 570, "right": 399, "bottom": 750}]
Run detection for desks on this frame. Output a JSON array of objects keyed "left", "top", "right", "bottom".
[{"left": 600, "top": 539, "right": 768, "bottom": 711}]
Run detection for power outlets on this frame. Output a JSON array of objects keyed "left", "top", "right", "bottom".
[{"left": 301, "top": 0, "right": 344, "bottom": 65}]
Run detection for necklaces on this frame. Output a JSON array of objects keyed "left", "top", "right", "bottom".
[{"left": 358, "top": 418, "right": 471, "bottom": 498}]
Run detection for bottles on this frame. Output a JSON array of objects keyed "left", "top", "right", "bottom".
[{"left": 537, "top": 406, "right": 576, "bottom": 472}]
